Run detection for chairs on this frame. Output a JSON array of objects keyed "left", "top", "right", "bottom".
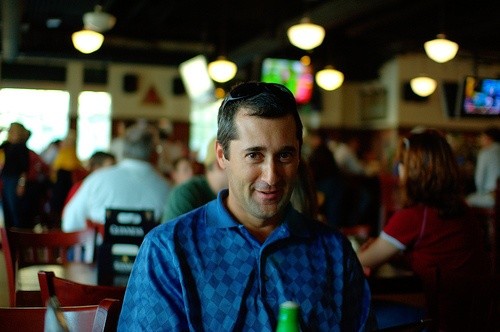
[{"left": 0, "top": 208, "right": 160, "bottom": 332}]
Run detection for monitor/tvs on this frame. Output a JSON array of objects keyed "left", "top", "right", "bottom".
[
  {"left": 257, "top": 53, "right": 321, "bottom": 106},
  {"left": 459, "top": 75, "right": 500, "bottom": 118}
]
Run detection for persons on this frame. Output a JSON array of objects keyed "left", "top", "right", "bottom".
[
  {"left": 116, "top": 82, "right": 374, "bottom": 332},
  {"left": 290, "top": 126, "right": 500, "bottom": 332},
  {"left": 0, "top": 82, "right": 229, "bottom": 332}
]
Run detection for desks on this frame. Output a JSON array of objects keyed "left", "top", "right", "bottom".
[
  {"left": 336, "top": 226, "right": 432, "bottom": 332},
  {"left": 15, "top": 261, "right": 137, "bottom": 291}
]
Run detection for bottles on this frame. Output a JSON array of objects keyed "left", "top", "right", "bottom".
[{"left": 275, "top": 301, "right": 301, "bottom": 332}]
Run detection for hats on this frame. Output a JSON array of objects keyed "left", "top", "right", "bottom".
[
  {"left": 113, "top": 123, "right": 159, "bottom": 160},
  {"left": 7, "top": 122, "right": 31, "bottom": 145}
]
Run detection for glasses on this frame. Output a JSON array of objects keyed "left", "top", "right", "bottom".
[{"left": 221, "top": 82, "right": 297, "bottom": 112}]
